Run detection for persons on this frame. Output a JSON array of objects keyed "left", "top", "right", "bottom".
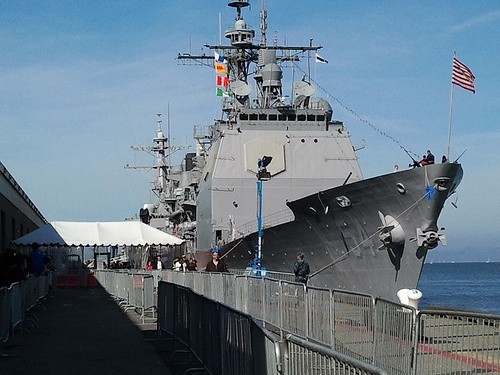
[
  {"left": 4, "top": 241, "right": 45, "bottom": 279},
  {"left": 173, "top": 254, "right": 198, "bottom": 274},
  {"left": 143, "top": 255, "right": 165, "bottom": 270},
  {"left": 205, "top": 251, "right": 230, "bottom": 274},
  {"left": 293, "top": 251, "right": 310, "bottom": 292},
  {"left": 420, "top": 150, "right": 435, "bottom": 165}
]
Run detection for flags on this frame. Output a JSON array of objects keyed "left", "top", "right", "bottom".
[
  {"left": 214, "top": 51, "right": 228, "bottom": 64},
  {"left": 216, "top": 75, "right": 230, "bottom": 89},
  {"left": 215, "top": 62, "right": 228, "bottom": 74},
  {"left": 216, "top": 87, "right": 231, "bottom": 98},
  {"left": 314, "top": 52, "right": 329, "bottom": 64},
  {"left": 451, "top": 56, "right": 476, "bottom": 95}
]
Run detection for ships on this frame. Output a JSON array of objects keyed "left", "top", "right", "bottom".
[{"left": 124, "top": 0, "right": 465, "bottom": 314}]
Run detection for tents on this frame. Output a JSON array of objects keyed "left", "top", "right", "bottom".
[{"left": 9, "top": 220, "right": 185, "bottom": 247}]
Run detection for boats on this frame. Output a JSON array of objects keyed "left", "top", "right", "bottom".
[
  {"left": 486, "top": 258, "right": 490, "bottom": 264},
  {"left": 430, "top": 261, "right": 433, "bottom": 265}
]
